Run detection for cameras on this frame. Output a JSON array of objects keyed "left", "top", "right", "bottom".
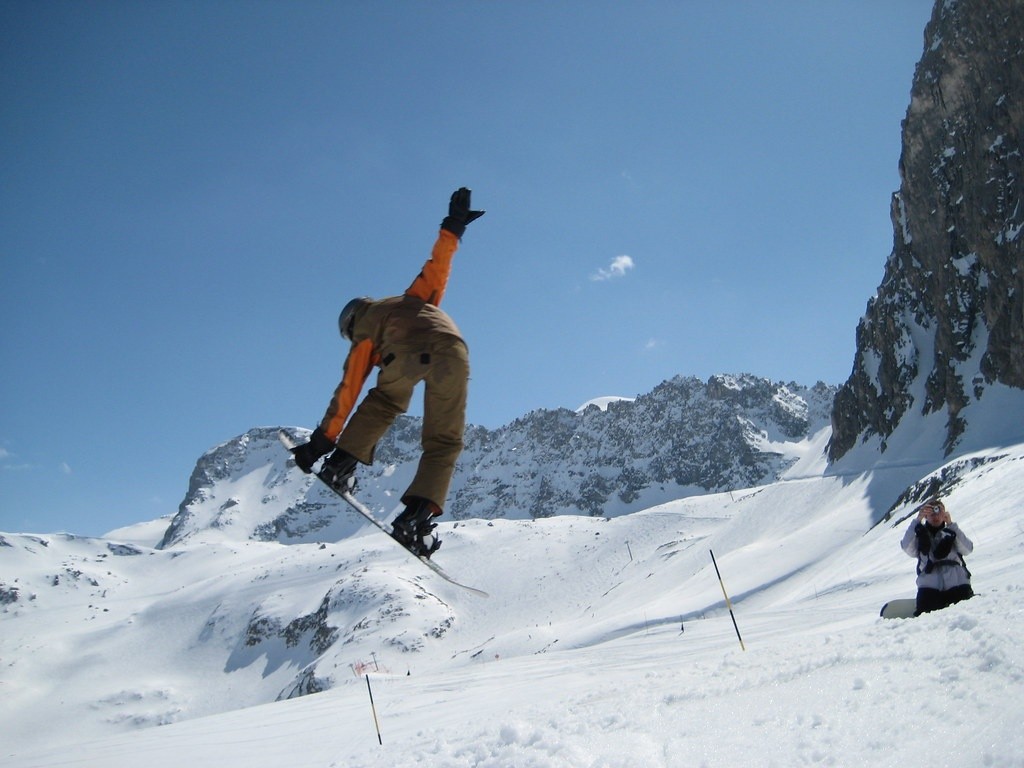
[{"left": 932, "top": 506, "right": 940, "bottom": 513}]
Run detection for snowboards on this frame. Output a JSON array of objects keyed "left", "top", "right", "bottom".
[
  {"left": 278, "top": 428, "right": 490, "bottom": 600},
  {"left": 880, "top": 594, "right": 980, "bottom": 620}
]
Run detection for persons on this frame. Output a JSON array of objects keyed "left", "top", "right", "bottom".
[
  {"left": 901, "top": 501, "right": 975, "bottom": 617},
  {"left": 288, "top": 187, "right": 486, "bottom": 560}
]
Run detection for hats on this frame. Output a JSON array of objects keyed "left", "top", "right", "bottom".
[{"left": 339, "top": 296, "right": 368, "bottom": 341}]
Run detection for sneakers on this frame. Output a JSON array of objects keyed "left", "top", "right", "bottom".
[
  {"left": 391, "top": 499, "right": 440, "bottom": 556},
  {"left": 319, "top": 449, "right": 362, "bottom": 496}
]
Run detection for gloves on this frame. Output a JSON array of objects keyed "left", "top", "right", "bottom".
[
  {"left": 289, "top": 427, "right": 335, "bottom": 474},
  {"left": 915, "top": 524, "right": 930, "bottom": 555},
  {"left": 934, "top": 535, "right": 953, "bottom": 559},
  {"left": 441, "top": 187, "right": 486, "bottom": 239}
]
